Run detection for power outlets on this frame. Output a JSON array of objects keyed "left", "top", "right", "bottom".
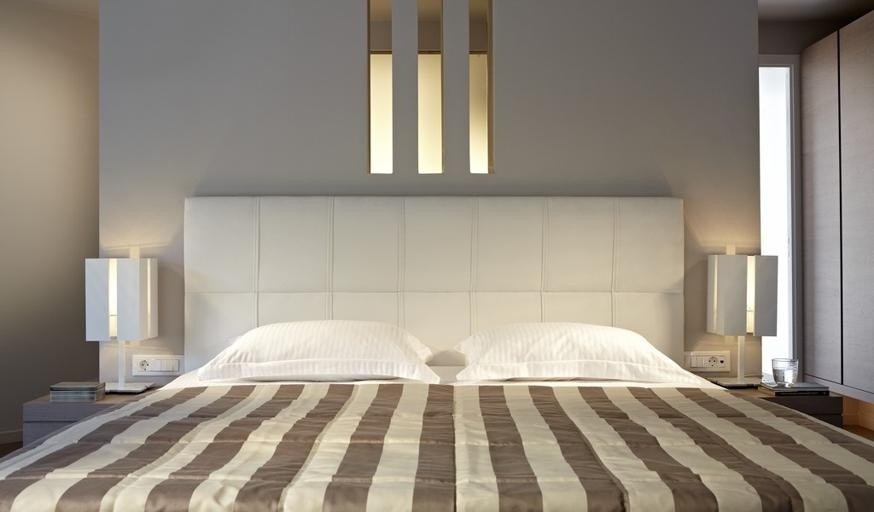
[
  {"left": 133, "top": 354, "right": 186, "bottom": 376},
  {"left": 684, "top": 349, "right": 730, "bottom": 373}
]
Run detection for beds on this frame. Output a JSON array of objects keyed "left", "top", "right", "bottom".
[{"left": 0, "top": 193, "right": 872, "bottom": 512}]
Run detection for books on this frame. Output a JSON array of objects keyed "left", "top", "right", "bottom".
[{"left": 757, "top": 381, "right": 830, "bottom": 398}]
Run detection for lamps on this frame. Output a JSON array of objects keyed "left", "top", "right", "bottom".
[
  {"left": 706, "top": 253, "right": 779, "bottom": 388},
  {"left": 82, "top": 258, "right": 162, "bottom": 395}
]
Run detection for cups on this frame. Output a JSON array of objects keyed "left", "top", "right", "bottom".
[{"left": 772, "top": 358, "right": 799, "bottom": 387}]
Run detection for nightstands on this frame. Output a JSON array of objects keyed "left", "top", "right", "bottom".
[
  {"left": 22, "top": 389, "right": 155, "bottom": 456},
  {"left": 731, "top": 387, "right": 843, "bottom": 430}
]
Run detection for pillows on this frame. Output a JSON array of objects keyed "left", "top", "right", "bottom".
[
  {"left": 199, "top": 319, "right": 440, "bottom": 387},
  {"left": 456, "top": 319, "right": 703, "bottom": 386}
]
